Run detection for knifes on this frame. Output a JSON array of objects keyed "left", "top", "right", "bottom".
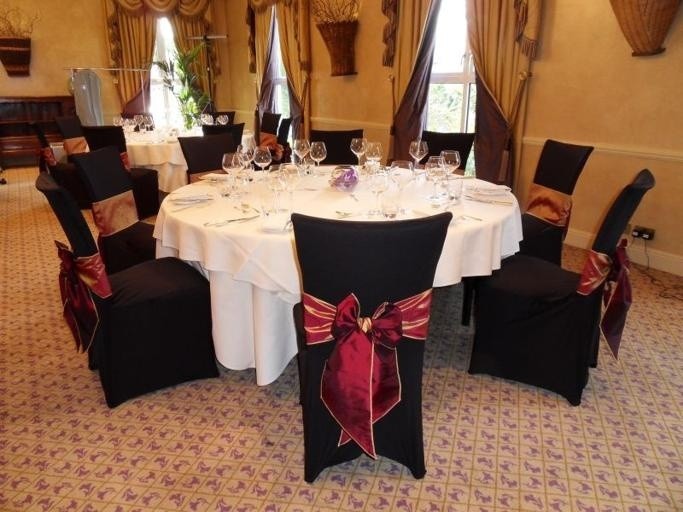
[
  {"left": 203, "top": 215, "right": 260, "bottom": 227},
  {"left": 465, "top": 194, "right": 514, "bottom": 206},
  {"left": 167, "top": 196, "right": 214, "bottom": 202}
]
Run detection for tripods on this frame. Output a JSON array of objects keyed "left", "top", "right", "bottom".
[{"left": 201, "top": 41, "right": 217, "bottom": 113}]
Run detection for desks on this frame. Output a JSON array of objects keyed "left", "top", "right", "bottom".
[{"left": 152, "top": 163, "right": 524, "bottom": 386}]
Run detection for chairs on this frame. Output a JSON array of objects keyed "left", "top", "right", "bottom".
[
  {"left": 467, "top": 168, "right": 655, "bottom": 407},
  {"left": 26, "top": 111, "right": 655, "bottom": 276},
  {"left": 291, "top": 213, "right": 454, "bottom": 483},
  {"left": 35, "top": 172, "right": 219, "bottom": 409},
  {"left": 460, "top": 138, "right": 595, "bottom": 327}
]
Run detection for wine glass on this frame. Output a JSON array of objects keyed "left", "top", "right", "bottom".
[
  {"left": 219, "top": 138, "right": 464, "bottom": 221},
  {"left": 112, "top": 112, "right": 230, "bottom": 144}
]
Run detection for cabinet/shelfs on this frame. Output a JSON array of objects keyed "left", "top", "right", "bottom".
[{"left": 0, "top": 95, "right": 78, "bottom": 168}]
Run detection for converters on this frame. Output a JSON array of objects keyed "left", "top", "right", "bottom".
[
  {"left": 631, "top": 228, "right": 644, "bottom": 238},
  {"left": 641, "top": 228, "right": 654, "bottom": 240}
]
